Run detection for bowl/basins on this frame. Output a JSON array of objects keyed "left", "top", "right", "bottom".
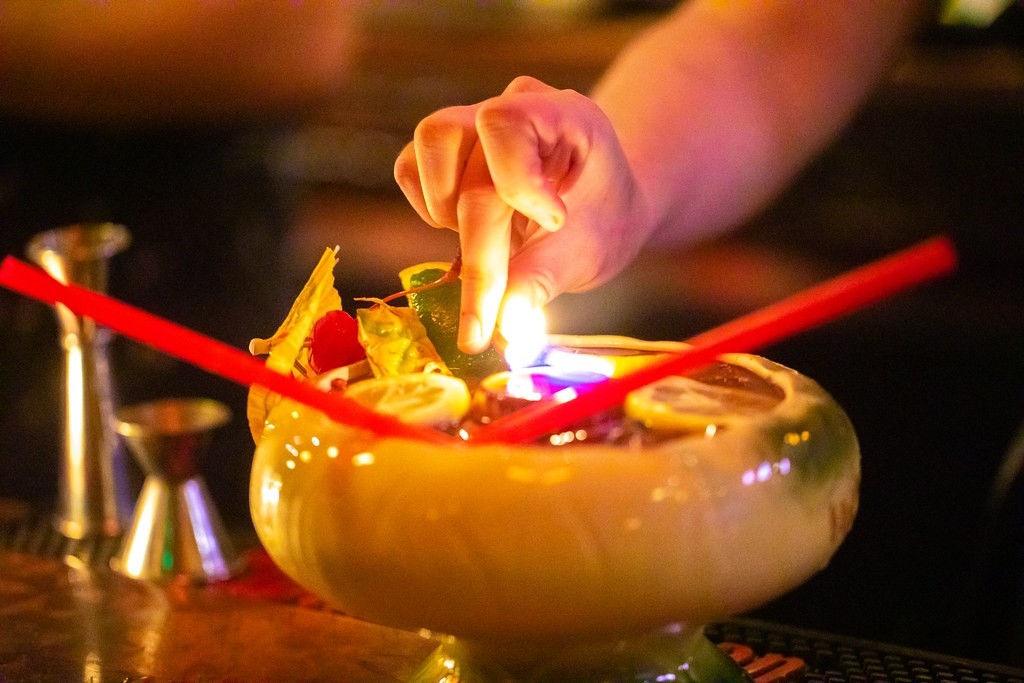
[{"left": 247, "top": 332, "right": 861, "bottom": 683}]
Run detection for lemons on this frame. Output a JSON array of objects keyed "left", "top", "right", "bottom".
[
  {"left": 623, "top": 376, "right": 781, "bottom": 434},
  {"left": 340, "top": 371, "right": 472, "bottom": 429},
  {"left": 397, "top": 261, "right": 510, "bottom": 386}
]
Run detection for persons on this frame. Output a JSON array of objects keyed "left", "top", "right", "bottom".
[{"left": 392, "top": 1, "right": 913, "bottom": 355}]
